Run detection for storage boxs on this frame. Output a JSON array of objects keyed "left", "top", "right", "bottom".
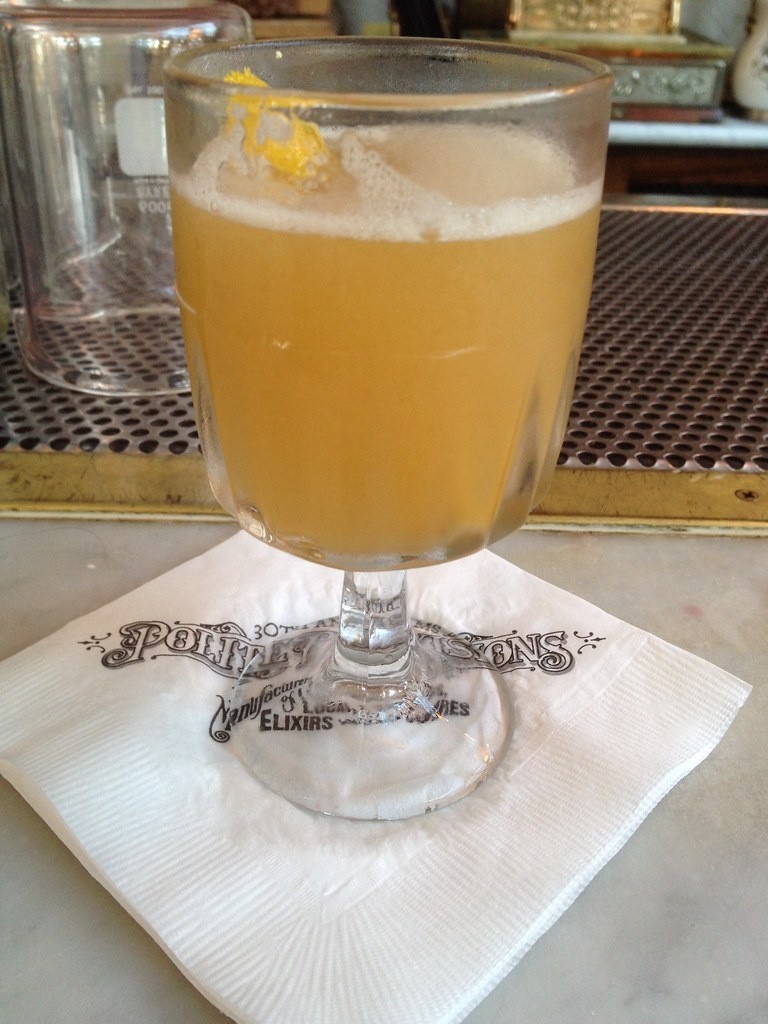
[{"left": 503, "top": 19, "right": 736, "bottom": 123}]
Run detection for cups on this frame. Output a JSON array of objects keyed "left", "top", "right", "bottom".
[{"left": 0, "top": 0, "right": 257, "bottom": 398}]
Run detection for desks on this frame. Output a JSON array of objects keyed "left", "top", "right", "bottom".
[{"left": 604, "top": 114, "right": 767, "bottom": 194}]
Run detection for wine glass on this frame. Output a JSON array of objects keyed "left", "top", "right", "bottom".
[{"left": 161, "top": 34, "right": 612, "bottom": 818}]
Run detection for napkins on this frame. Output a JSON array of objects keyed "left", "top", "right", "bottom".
[{"left": 2, "top": 526, "right": 755, "bottom": 1022}]
